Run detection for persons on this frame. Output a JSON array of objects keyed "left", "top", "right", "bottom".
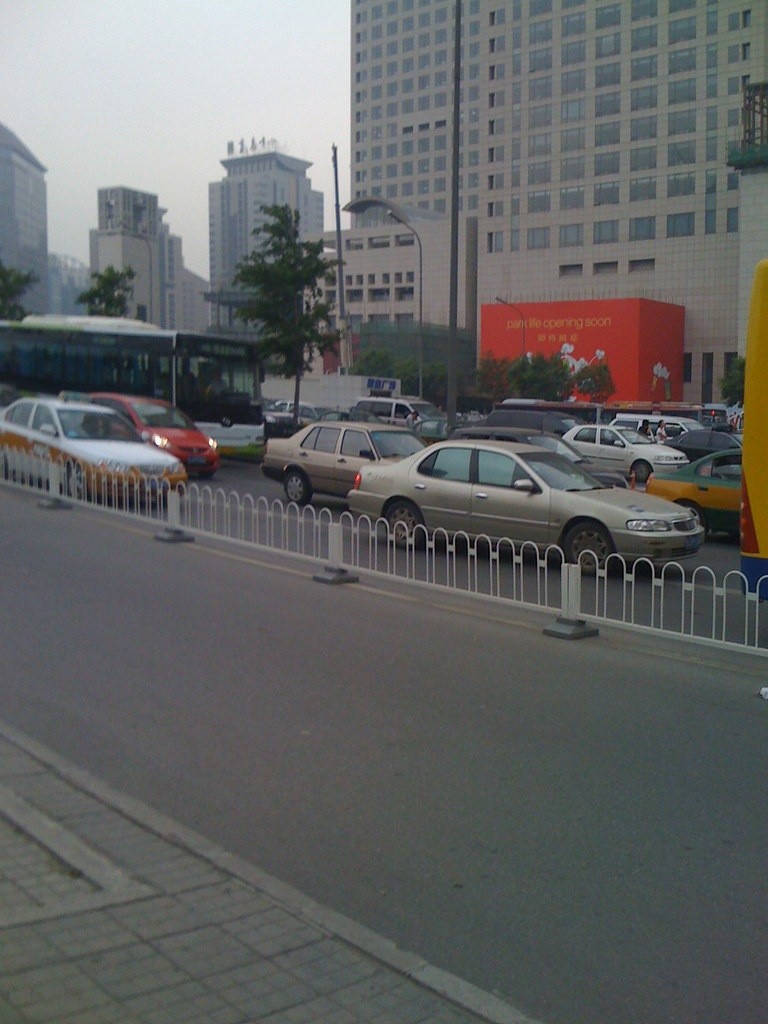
[
  {"left": 639, "top": 419, "right": 666, "bottom": 444},
  {"left": 405, "top": 411, "right": 420, "bottom": 428},
  {"left": 74, "top": 413, "right": 101, "bottom": 439},
  {"left": 209, "top": 368, "right": 229, "bottom": 393}
]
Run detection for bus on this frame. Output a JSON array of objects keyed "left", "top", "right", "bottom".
[
  {"left": 740, "top": 258, "right": 768, "bottom": 603},
  {"left": 0, "top": 315, "right": 268, "bottom": 461}
]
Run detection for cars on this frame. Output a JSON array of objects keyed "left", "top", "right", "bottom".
[
  {"left": 645, "top": 445, "right": 742, "bottom": 543},
  {"left": 261, "top": 393, "right": 742, "bottom": 488},
  {"left": 68, "top": 391, "right": 220, "bottom": 480},
  {"left": 1, "top": 397, "right": 189, "bottom": 509},
  {"left": 259, "top": 420, "right": 428, "bottom": 506},
  {"left": 346, "top": 438, "right": 706, "bottom": 576}
]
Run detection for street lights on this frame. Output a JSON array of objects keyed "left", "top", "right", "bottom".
[
  {"left": 386, "top": 208, "right": 424, "bottom": 399},
  {"left": 495, "top": 297, "right": 526, "bottom": 397}
]
